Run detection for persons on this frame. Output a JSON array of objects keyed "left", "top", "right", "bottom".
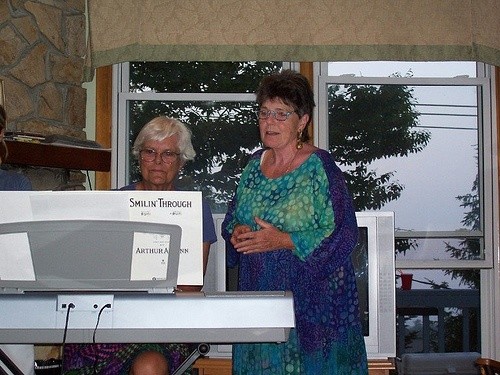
[
  {"left": 61, "top": 116, "right": 217, "bottom": 374},
  {"left": 221, "top": 70, "right": 369, "bottom": 375},
  {"left": 0, "top": 102, "right": 35, "bottom": 191}
]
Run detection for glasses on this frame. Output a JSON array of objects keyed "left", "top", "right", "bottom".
[
  {"left": 254, "top": 109, "right": 296, "bottom": 121},
  {"left": 137, "top": 148, "right": 182, "bottom": 164}
]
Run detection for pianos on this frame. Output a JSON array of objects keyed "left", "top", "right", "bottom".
[{"left": 0, "top": 290, "right": 296, "bottom": 375}]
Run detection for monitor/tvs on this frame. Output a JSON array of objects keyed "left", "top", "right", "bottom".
[{"left": 204, "top": 211, "right": 396, "bottom": 360}]
